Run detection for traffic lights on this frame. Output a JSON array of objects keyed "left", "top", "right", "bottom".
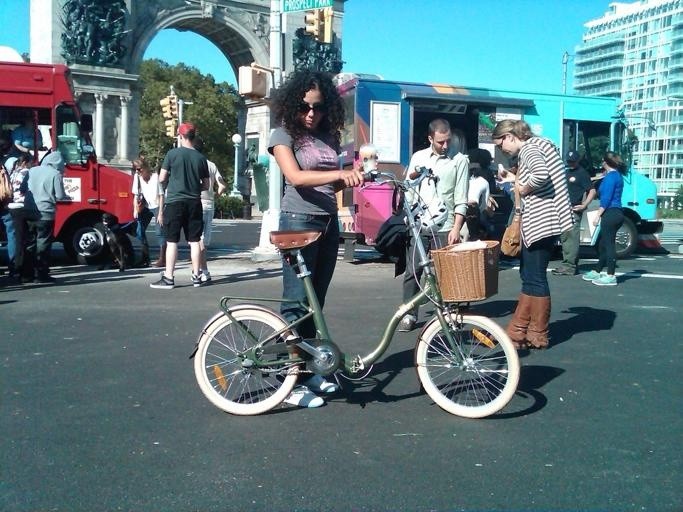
[
  {"left": 314, "top": 7, "right": 334, "bottom": 44},
  {"left": 304, "top": 7, "right": 320, "bottom": 38},
  {"left": 160, "top": 96, "right": 170, "bottom": 118},
  {"left": 164, "top": 119, "right": 177, "bottom": 137},
  {"left": 168, "top": 95, "right": 178, "bottom": 117}
]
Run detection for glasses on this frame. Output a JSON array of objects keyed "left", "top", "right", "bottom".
[
  {"left": 496, "top": 138, "right": 506, "bottom": 152},
  {"left": 298, "top": 102, "right": 323, "bottom": 115}
]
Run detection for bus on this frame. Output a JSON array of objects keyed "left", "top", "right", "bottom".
[
  {"left": 1, "top": 59, "right": 139, "bottom": 265},
  {"left": 330, "top": 73, "right": 666, "bottom": 262}
]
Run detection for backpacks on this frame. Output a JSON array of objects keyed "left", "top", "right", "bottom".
[{"left": 499, "top": 216, "right": 524, "bottom": 258}]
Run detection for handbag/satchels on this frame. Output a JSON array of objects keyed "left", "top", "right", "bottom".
[
  {"left": 137, "top": 193, "right": 146, "bottom": 216},
  {"left": 374, "top": 185, "right": 409, "bottom": 260},
  {"left": 2, "top": 164, "right": 14, "bottom": 205}
]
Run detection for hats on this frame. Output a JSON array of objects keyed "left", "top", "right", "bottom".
[
  {"left": 565, "top": 150, "right": 580, "bottom": 162},
  {"left": 177, "top": 123, "right": 195, "bottom": 137}
]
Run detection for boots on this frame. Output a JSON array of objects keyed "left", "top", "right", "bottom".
[
  {"left": 155, "top": 244, "right": 166, "bottom": 268},
  {"left": 524, "top": 295, "right": 550, "bottom": 349},
  {"left": 136, "top": 243, "right": 151, "bottom": 268},
  {"left": 505, "top": 290, "right": 532, "bottom": 352}
]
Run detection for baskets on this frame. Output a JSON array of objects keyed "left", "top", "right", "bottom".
[{"left": 428, "top": 239, "right": 500, "bottom": 303}]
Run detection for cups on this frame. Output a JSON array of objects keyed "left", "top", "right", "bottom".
[{"left": 358, "top": 143, "right": 381, "bottom": 175}]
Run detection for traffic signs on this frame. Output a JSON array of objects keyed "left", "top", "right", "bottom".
[{"left": 283, "top": 0, "right": 334, "bottom": 14}]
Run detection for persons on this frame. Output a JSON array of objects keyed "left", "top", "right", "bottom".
[
  {"left": 489, "top": 119, "right": 577, "bottom": 349},
  {"left": 150, "top": 123, "right": 210, "bottom": 289},
  {"left": 267, "top": 70, "right": 365, "bottom": 408},
  {"left": 397, "top": 119, "right": 470, "bottom": 332},
  {"left": 0, "top": 111, "right": 66, "bottom": 283},
  {"left": 466, "top": 151, "right": 628, "bottom": 286},
  {"left": 131, "top": 158, "right": 168, "bottom": 267},
  {"left": 191, "top": 139, "right": 228, "bottom": 285}
]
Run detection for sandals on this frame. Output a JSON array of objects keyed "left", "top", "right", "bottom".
[
  {"left": 304, "top": 373, "right": 340, "bottom": 395},
  {"left": 282, "top": 384, "right": 325, "bottom": 408}
]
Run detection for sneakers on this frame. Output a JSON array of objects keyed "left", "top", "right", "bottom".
[
  {"left": 550, "top": 266, "right": 569, "bottom": 276},
  {"left": 149, "top": 272, "right": 175, "bottom": 290},
  {"left": 190, "top": 270, "right": 211, "bottom": 288},
  {"left": 582, "top": 271, "right": 600, "bottom": 282},
  {"left": 591, "top": 275, "right": 618, "bottom": 286}
]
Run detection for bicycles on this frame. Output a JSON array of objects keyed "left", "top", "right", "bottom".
[{"left": 182, "top": 165, "right": 526, "bottom": 421}]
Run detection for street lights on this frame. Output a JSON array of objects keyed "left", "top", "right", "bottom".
[{"left": 230, "top": 132, "right": 243, "bottom": 196}]
